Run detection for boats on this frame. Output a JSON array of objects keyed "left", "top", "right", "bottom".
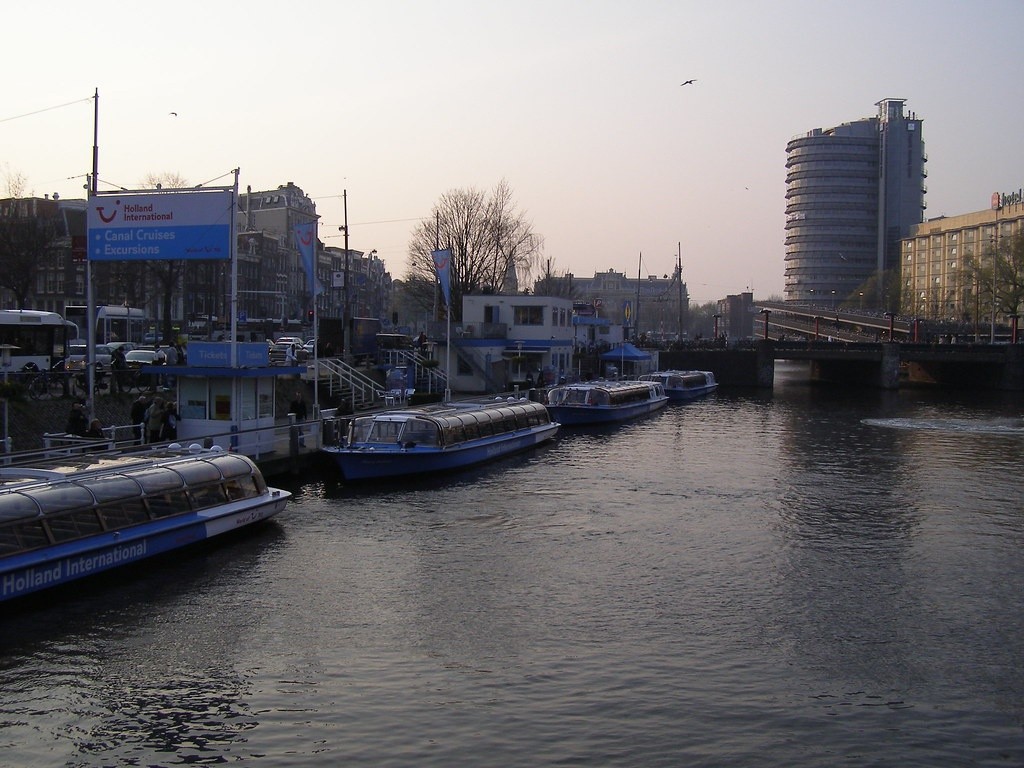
[
  {"left": 320, "top": 396, "right": 563, "bottom": 484},
  {"left": 544, "top": 380, "right": 670, "bottom": 430},
  {"left": 0, "top": 441, "right": 294, "bottom": 607},
  {"left": 636, "top": 370, "right": 720, "bottom": 403}
]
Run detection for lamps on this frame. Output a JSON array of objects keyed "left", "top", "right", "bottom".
[{"left": 551, "top": 335, "right": 556, "bottom": 339}]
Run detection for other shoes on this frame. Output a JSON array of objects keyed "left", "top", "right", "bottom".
[{"left": 299, "top": 443, "right": 306, "bottom": 448}]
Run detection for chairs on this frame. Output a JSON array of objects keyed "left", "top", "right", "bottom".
[
  {"left": 404, "top": 388, "right": 415, "bottom": 403},
  {"left": 455, "top": 327, "right": 471, "bottom": 337},
  {"left": 383, "top": 392, "right": 396, "bottom": 407},
  {"left": 376, "top": 389, "right": 388, "bottom": 406},
  {"left": 391, "top": 389, "right": 402, "bottom": 404}
]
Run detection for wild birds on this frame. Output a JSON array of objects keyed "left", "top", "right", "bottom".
[{"left": 681, "top": 80, "right": 697, "bottom": 86}]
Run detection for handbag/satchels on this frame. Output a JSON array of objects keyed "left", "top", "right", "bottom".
[{"left": 169, "top": 414, "right": 178, "bottom": 428}]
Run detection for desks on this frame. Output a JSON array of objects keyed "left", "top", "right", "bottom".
[{"left": 384, "top": 392, "right": 402, "bottom": 405}]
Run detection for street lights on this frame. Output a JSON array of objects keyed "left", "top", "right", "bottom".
[
  {"left": 831, "top": 290, "right": 835, "bottom": 311},
  {"left": 988, "top": 234, "right": 1003, "bottom": 345},
  {"left": 859, "top": 292, "right": 864, "bottom": 315}
]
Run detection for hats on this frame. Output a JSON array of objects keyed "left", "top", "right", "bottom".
[{"left": 139, "top": 396, "right": 146, "bottom": 400}]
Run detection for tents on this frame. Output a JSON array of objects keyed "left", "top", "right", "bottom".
[{"left": 598, "top": 342, "right": 652, "bottom": 375}]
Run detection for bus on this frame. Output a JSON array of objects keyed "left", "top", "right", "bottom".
[
  {"left": 0, "top": 309, "right": 80, "bottom": 384},
  {"left": 64, "top": 305, "right": 145, "bottom": 345}
]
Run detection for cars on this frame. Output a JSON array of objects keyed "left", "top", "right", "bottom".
[
  {"left": 70, "top": 341, "right": 170, "bottom": 367},
  {"left": 266, "top": 336, "right": 315, "bottom": 357}
]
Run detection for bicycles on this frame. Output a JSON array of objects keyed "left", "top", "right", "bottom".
[
  {"left": 14, "top": 360, "right": 108, "bottom": 402},
  {"left": 117, "top": 364, "right": 152, "bottom": 394}
]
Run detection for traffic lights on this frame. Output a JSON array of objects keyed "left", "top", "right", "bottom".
[{"left": 308, "top": 310, "right": 314, "bottom": 323}]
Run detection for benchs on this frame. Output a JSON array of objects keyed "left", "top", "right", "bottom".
[
  {"left": 391, "top": 422, "right": 527, "bottom": 445},
  {"left": 0, "top": 496, "right": 219, "bottom": 556}
]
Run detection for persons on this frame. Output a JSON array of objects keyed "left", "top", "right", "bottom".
[
  {"left": 68, "top": 401, "right": 89, "bottom": 436},
  {"left": 155, "top": 344, "right": 165, "bottom": 386},
  {"left": 145, "top": 393, "right": 182, "bottom": 442},
  {"left": 130, "top": 396, "right": 147, "bottom": 444},
  {"left": 313, "top": 339, "right": 325, "bottom": 358},
  {"left": 526, "top": 371, "right": 533, "bottom": 389},
  {"left": 418, "top": 331, "right": 427, "bottom": 352},
  {"left": 538, "top": 372, "right": 543, "bottom": 383},
  {"left": 288, "top": 391, "right": 308, "bottom": 448},
  {"left": 167, "top": 342, "right": 178, "bottom": 366},
  {"left": 88, "top": 418, "right": 106, "bottom": 451},
  {"left": 337, "top": 399, "right": 347, "bottom": 412},
  {"left": 110, "top": 346, "right": 124, "bottom": 394},
  {"left": 325, "top": 342, "right": 335, "bottom": 357}
]
